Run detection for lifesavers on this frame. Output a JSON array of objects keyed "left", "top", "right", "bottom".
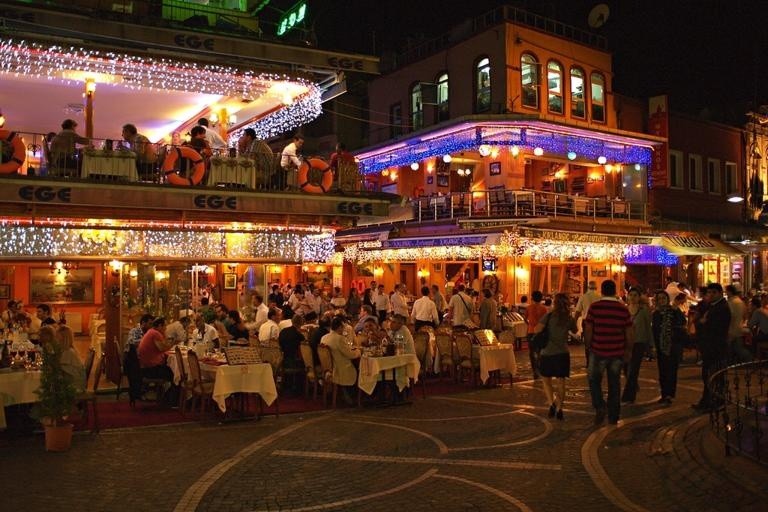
[
  {"left": 299, "top": 159, "right": 334, "bottom": 193},
  {"left": 164, "top": 147, "right": 205, "bottom": 186},
  {"left": 0, "top": 129, "right": 26, "bottom": 173}
]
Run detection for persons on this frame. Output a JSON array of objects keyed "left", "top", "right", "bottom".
[
  {"left": 1, "top": 301, "right": 86, "bottom": 395},
  {"left": 330, "top": 143, "right": 353, "bottom": 180},
  {"left": 524, "top": 291, "right": 548, "bottom": 379},
  {"left": 586, "top": 279, "right": 635, "bottom": 424},
  {"left": 280, "top": 134, "right": 305, "bottom": 170},
  {"left": 621, "top": 276, "right": 768, "bottom": 369},
  {"left": 692, "top": 283, "right": 731, "bottom": 413},
  {"left": 1, "top": 116, "right": 279, "bottom": 191},
  {"left": 119, "top": 277, "right": 497, "bottom": 407},
  {"left": 652, "top": 291, "right": 686, "bottom": 407},
  {"left": 567, "top": 295, "right": 579, "bottom": 344},
  {"left": 516, "top": 296, "right": 530, "bottom": 313},
  {"left": 534, "top": 293, "right": 577, "bottom": 419},
  {"left": 540, "top": 294, "right": 555, "bottom": 312},
  {"left": 573, "top": 281, "right": 600, "bottom": 369},
  {"left": 622, "top": 288, "right": 651, "bottom": 403}
]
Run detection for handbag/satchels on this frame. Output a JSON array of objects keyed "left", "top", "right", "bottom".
[{"left": 531, "top": 329, "right": 548, "bottom": 352}]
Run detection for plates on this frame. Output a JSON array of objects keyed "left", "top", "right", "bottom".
[{"left": 364, "top": 354, "right": 375, "bottom": 357}]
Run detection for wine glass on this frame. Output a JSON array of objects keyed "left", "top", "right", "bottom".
[{"left": 10, "top": 345, "right": 35, "bottom": 374}]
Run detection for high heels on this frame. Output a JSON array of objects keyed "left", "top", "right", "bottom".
[
  {"left": 547, "top": 403, "right": 555, "bottom": 419},
  {"left": 556, "top": 409, "right": 562, "bottom": 421}
]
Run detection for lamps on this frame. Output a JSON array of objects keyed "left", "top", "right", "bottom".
[{"left": 382, "top": 145, "right": 641, "bottom": 182}]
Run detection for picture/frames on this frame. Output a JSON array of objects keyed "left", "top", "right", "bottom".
[{"left": 223, "top": 273, "right": 238, "bottom": 290}]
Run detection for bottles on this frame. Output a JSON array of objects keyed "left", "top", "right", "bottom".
[
  {"left": 381, "top": 334, "right": 387, "bottom": 355},
  {"left": 205, "top": 340, "right": 211, "bottom": 360},
  {"left": 3, "top": 340, "right": 11, "bottom": 367},
  {"left": 396, "top": 330, "right": 404, "bottom": 355}
]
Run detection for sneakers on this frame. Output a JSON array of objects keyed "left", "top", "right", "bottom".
[{"left": 594, "top": 405, "right": 606, "bottom": 425}]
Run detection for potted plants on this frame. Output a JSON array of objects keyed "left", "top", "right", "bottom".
[{"left": 28, "top": 335, "right": 77, "bottom": 453}]
